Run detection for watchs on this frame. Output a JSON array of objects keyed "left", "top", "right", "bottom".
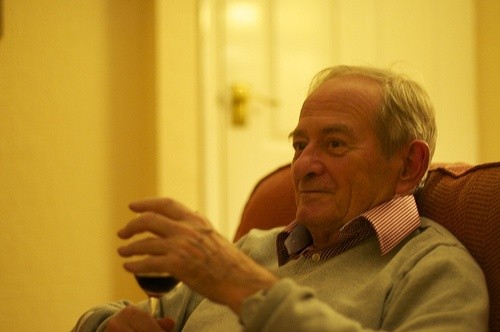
[{"left": 238, "top": 288, "right": 269, "bottom": 326}]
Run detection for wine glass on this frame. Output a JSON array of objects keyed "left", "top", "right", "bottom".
[{"left": 130, "top": 215, "right": 184, "bottom": 319}]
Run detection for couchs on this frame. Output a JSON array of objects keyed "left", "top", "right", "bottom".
[{"left": 233, "top": 158, "right": 500, "bottom": 332}]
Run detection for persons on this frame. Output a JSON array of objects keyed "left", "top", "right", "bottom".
[{"left": 72, "top": 65, "right": 489, "bottom": 332}]
